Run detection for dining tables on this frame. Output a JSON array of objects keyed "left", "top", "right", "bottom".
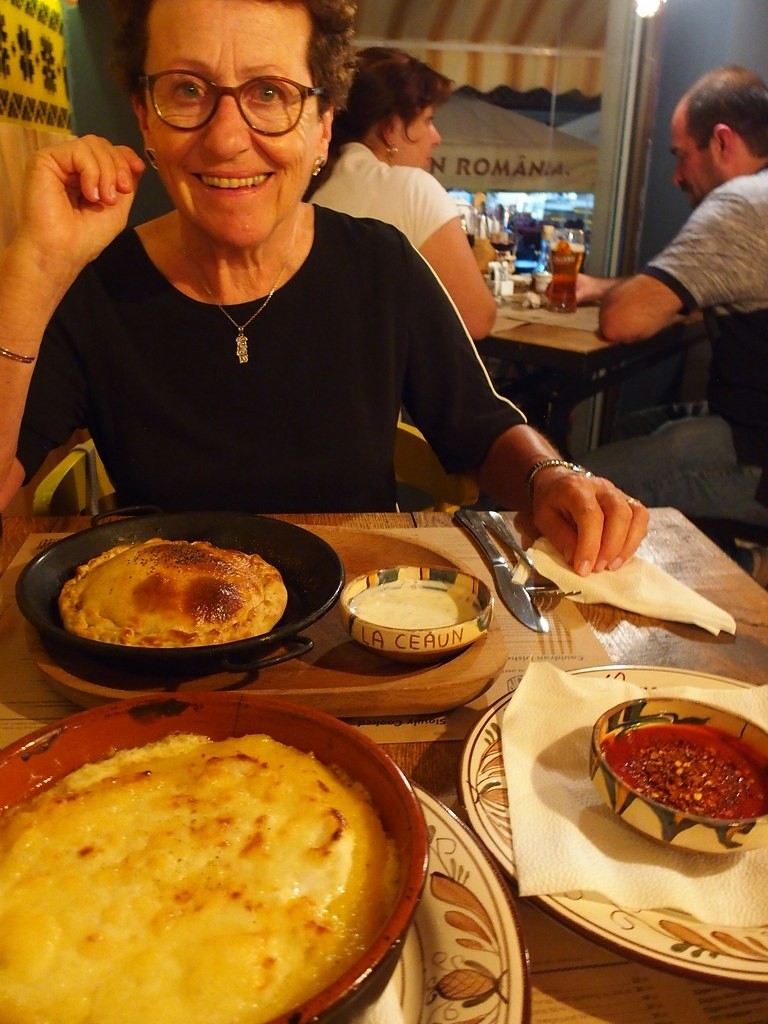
[{"left": 0, "top": 506, "right": 768, "bottom": 1024}]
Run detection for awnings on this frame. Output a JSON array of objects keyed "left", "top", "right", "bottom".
[{"left": 350, "top": 0, "right": 612, "bottom": 96}]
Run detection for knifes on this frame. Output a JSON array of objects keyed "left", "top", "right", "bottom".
[{"left": 454, "top": 508, "right": 543, "bottom": 634}]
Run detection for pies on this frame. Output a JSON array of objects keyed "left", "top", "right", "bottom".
[{"left": 58, "top": 537, "right": 287, "bottom": 648}]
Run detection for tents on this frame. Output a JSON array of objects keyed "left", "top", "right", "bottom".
[{"left": 425, "top": 92, "right": 599, "bottom": 193}]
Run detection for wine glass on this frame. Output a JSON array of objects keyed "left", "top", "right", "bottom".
[{"left": 490, "top": 212, "right": 517, "bottom": 262}]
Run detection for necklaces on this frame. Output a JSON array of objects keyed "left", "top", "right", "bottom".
[{"left": 183, "top": 214, "right": 301, "bottom": 365}]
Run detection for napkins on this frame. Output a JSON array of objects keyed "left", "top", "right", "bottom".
[
  {"left": 502, "top": 661, "right": 768, "bottom": 928},
  {"left": 511, "top": 539, "right": 736, "bottom": 637}
]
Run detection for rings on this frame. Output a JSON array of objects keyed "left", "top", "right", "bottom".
[{"left": 627, "top": 498, "right": 640, "bottom": 503}]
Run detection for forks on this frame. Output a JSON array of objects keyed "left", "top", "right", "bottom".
[{"left": 480, "top": 510, "right": 581, "bottom": 597}]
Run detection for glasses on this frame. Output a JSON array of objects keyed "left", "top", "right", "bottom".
[{"left": 136, "top": 68, "right": 325, "bottom": 138}]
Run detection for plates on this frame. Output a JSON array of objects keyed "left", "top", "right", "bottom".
[
  {"left": 325, "top": 779, "right": 533, "bottom": 1024},
  {"left": 457, "top": 664, "right": 768, "bottom": 990}
]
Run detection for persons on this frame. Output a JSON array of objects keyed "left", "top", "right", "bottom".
[
  {"left": 0, "top": 0, "right": 649, "bottom": 578},
  {"left": 308, "top": 46, "right": 496, "bottom": 340},
  {"left": 547, "top": 64, "right": 768, "bottom": 589}
]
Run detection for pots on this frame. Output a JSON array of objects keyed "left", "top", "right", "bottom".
[{"left": 14, "top": 501, "right": 345, "bottom": 683}]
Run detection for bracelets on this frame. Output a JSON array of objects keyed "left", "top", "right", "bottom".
[
  {"left": 526, "top": 459, "right": 594, "bottom": 503},
  {"left": 0, "top": 347, "right": 35, "bottom": 363}
]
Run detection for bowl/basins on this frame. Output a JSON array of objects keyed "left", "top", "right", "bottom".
[
  {"left": 339, "top": 564, "right": 493, "bottom": 665},
  {"left": 590, "top": 696, "right": 768, "bottom": 855},
  {"left": 0, "top": 690, "right": 430, "bottom": 1024}
]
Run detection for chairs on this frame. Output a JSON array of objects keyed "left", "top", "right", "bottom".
[{"left": 30, "top": 422, "right": 481, "bottom": 520}]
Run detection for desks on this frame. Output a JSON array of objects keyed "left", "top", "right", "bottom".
[{"left": 485, "top": 275, "right": 709, "bottom": 458}]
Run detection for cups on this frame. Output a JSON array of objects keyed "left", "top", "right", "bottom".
[
  {"left": 458, "top": 204, "right": 475, "bottom": 248},
  {"left": 545, "top": 228, "right": 585, "bottom": 314}
]
